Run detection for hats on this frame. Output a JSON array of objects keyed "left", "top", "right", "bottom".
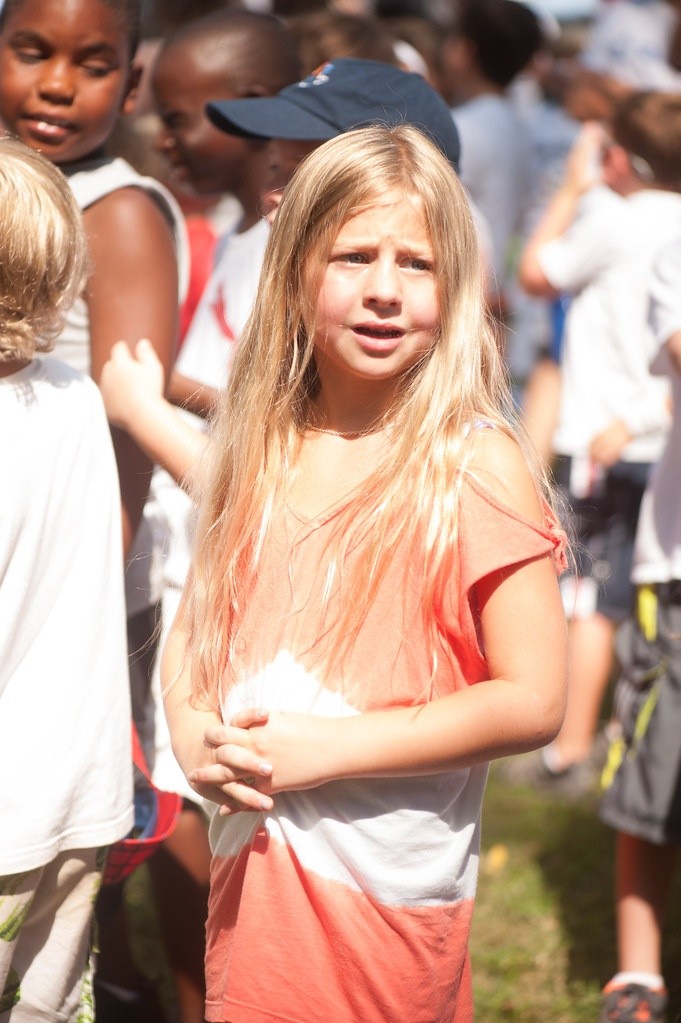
[{"left": 203, "top": 57, "right": 459, "bottom": 172}]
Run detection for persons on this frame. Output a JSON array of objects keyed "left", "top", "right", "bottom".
[
  {"left": 159, "top": 127, "right": 568, "bottom": 1023},
  {"left": 0, "top": 0, "right": 681, "bottom": 1023}
]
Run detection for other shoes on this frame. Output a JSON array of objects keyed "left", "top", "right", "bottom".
[
  {"left": 600, "top": 972, "right": 668, "bottom": 1023},
  {"left": 508, "top": 746, "right": 596, "bottom": 797}
]
